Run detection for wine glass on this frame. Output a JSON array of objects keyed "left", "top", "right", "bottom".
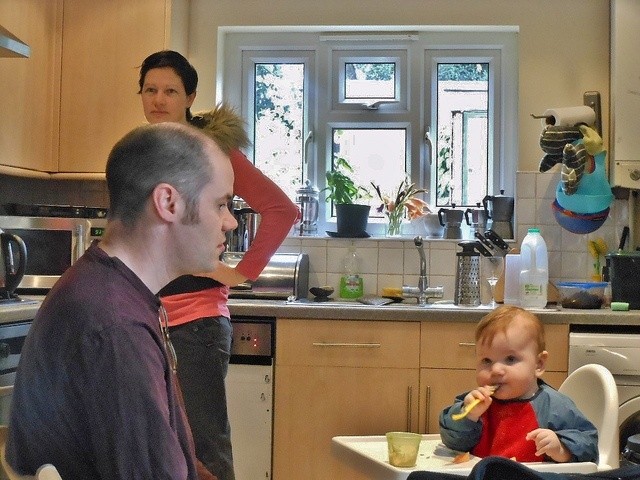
[{"left": 484, "top": 256, "right": 503, "bottom": 308}]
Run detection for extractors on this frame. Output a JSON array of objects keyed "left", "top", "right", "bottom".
[{"left": 0, "top": 22, "right": 33, "bottom": 58}]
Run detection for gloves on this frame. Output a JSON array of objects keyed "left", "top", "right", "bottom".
[
  {"left": 561, "top": 143, "right": 586, "bottom": 196},
  {"left": 538, "top": 124, "right": 583, "bottom": 174}
]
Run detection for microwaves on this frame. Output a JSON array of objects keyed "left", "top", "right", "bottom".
[{"left": 0, "top": 203, "right": 110, "bottom": 294}]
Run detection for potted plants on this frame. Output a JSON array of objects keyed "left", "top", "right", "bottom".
[{"left": 319, "top": 157, "right": 371, "bottom": 230}]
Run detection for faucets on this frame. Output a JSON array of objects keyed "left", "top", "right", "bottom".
[{"left": 403, "top": 236, "right": 443, "bottom": 304}]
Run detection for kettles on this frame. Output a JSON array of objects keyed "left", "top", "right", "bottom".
[{"left": 0, "top": 226, "right": 27, "bottom": 298}]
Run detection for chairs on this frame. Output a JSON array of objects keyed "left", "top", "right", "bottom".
[{"left": 332, "top": 362, "right": 620, "bottom": 480}]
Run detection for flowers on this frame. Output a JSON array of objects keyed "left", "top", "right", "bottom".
[{"left": 371, "top": 178, "right": 427, "bottom": 218}]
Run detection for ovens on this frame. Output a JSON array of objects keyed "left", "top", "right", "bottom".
[
  {"left": 2, "top": 322, "right": 36, "bottom": 429},
  {"left": 223, "top": 315, "right": 273, "bottom": 480}
]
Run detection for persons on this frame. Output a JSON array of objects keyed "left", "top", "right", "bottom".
[
  {"left": 4, "top": 121, "right": 238, "bottom": 479},
  {"left": 438, "top": 305, "right": 598, "bottom": 462},
  {"left": 132, "top": 50, "right": 300, "bottom": 479}
]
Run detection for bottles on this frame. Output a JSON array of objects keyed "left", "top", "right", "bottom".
[
  {"left": 483, "top": 190, "right": 513, "bottom": 238},
  {"left": 465, "top": 201, "right": 490, "bottom": 241},
  {"left": 296, "top": 179, "right": 320, "bottom": 236},
  {"left": 438, "top": 203, "right": 463, "bottom": 239}
]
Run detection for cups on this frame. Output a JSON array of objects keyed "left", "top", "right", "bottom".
[{"left": 386, "top": 431, "right": 423, "bottom": 467}]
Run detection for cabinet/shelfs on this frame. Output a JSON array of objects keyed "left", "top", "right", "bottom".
[
  {"left": 1, "top": 1, "right": 58, "bottom": 180},
  {"left": 274, "top": 306, "right": 568, "bottom": 480},
  {"left": 59, "top": 1, "right": 171, "bottom": 180}
]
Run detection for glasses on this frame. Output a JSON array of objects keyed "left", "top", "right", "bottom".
[{"left": 158, "top": 304, "right": 178, "bottom": 375}]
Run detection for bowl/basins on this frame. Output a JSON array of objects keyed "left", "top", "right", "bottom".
[{"left": 557, "top": 281, "right": 607, "bottom": 308}]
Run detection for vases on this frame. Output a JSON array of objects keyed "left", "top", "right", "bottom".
[{"left": 384, "top": 211, "right": 402, "bottom": 237}]
